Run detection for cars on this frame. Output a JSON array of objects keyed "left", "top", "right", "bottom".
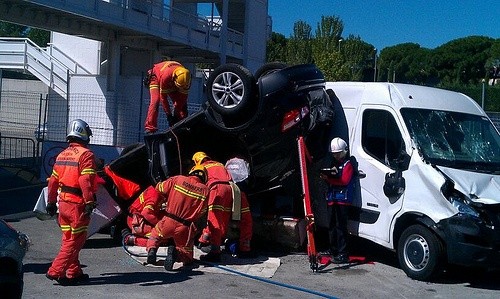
[{"left": 94, "top": 60, "right": 337, "bottom": 204}]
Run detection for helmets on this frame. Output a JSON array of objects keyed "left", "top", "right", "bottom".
[
  {"left": 328, "top": 137, "right": 348, "bottom": 153},
  {"left": 65, "top": 119, "right": 93, "bottom": 141},
  {"left": 189, "top": 165, "right": 208, "bottom": 182},
  {"left": 172, "top": 67, "right": 191, "bottom": 89},
  {"left": 192, "top": 151, "right": 209, "bottom": 165}
]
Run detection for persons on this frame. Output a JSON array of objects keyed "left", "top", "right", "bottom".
[
  {"left": 319, "top": 136, "right": 355, "bottom": 264},
  {"left": 125, "top": 152, "right": 255, "bottom": 271},
  {"left": 143, "top": 60, "right": 191, "bottom": 134},
  {"left": 45, "top": 119, "right": 98, "bottom": 286}
]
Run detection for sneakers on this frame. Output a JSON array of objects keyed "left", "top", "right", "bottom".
[
  {"left": 66, "top": 273, "right": 89, "bottom": 283},
  {"left": 46, "top": 272, "right": 68, "bottom": 285}
]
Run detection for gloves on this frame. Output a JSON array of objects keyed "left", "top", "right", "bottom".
[
  {"left": 167, "top": 112, "right": 177, "bottom": 126},
  {"left": 45, "top": 203, "right": 56, "bottom": 216},
  {"left": 81, "top": 204, "right": 95, "bottom": 218}
]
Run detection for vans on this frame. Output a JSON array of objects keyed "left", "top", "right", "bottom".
[{"left": 325, "top": 82, "right": 500, "bottom": 281}]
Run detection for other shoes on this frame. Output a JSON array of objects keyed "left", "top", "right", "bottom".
[
  {"left": 332, "top": 254, "right": 349, "bottom": 262},
  {"left": 164, "top": 245, "right": 176, "bottom": 271},
  {"left": 238, "top": 250, "right": 258, "bottom": 258},
  {"left": 147, "top": 249, "right": 156, "bottom": 264},
  {"left": 200, "top": 253, "right": 221, "bottom": 262},
  {"left": 121, "top": 227, "right": 131, "bottom": 245},
  {"left": 320, "top": 251, "right": 332, "bottom": 256}
]
